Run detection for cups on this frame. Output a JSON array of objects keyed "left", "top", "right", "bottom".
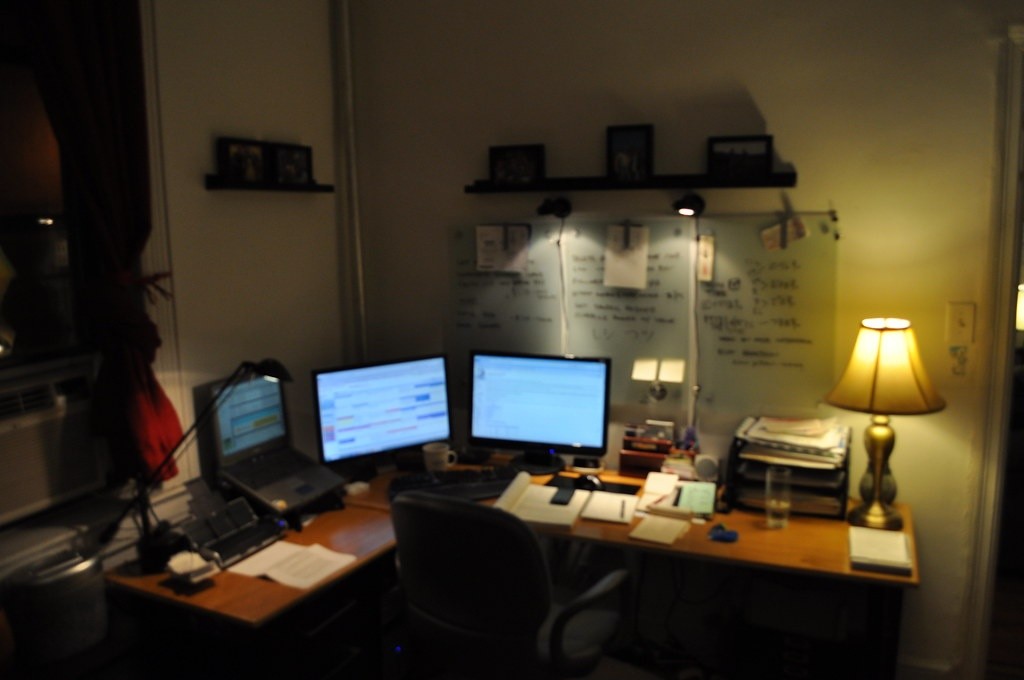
[
  {"left": 766, "top": 466, "right": 793, "bottom": 529},
  {"left": 422, "top": 443, "right": 457, "bottom": 473}
]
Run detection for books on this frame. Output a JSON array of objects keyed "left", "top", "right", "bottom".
[
  {"left": 849, "top": 525, "right": 912, "bottom": 577},
  {"left": 635, "top": 471, "right": 716, "bottom": 521},
  {"left": 580, "top": 490, "right": 639, "bottom": 524},
  {"left": 493, "top": 470, "right": 590, "bottom": 531}
]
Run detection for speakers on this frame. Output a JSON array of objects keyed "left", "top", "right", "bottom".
[{"left": 694, "top": 454, "right": 720, "bottom": 481}]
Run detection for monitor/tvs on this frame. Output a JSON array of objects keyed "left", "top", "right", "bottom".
[
  {"left": 310, "top": 354, "right": 454, "bottom": 481},
  {"left": 467, "top": 350, "right": 610, "bottom": 476}
]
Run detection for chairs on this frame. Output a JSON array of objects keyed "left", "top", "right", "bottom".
[{"left": 388, "top": 493, "right": 638, "bottom": 680}]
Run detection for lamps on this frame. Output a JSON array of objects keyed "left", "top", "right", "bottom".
[
  {"left": 824, "top": 316, "right": 947, "bottom": 530},
  {"left": 674, "top": 193, "right": 707, "bottom": 218},
  {"left": 534, "top": 191, "right": 587, "bottom": 221},
  {"left": 130, "top": 357, "right": 296, "bottom": 576}
]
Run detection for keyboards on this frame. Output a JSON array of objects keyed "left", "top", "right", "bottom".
[{"left": 388, "top": 467, "right": 520, "bottom": 503}]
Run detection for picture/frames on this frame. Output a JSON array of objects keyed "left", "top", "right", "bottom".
[
  {"left": 267, "top": 140, "right": 314, "bottom": 183},
  {"left": 214, "top": 135, "right": 267, "bottom": 178},
  {"left": 705, "top": 134, "right": 774, "bottom": 173},
  {"left": 605, "top": 124, "right": 655, "bottom": 179},
  {"left": 489, "top": 142, "right": 546, "bottom": 181}
]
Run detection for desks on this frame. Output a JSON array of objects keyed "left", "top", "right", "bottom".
[
  {"left": 102, "top": 505, "right": 396, "bottom": 680},
  {"left": 344, "top": 456, "right": 918, "bottom": 680}
]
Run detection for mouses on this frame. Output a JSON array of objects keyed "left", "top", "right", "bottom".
[{"left": 578, "top": 473, "right": 604, "bottom": 491}]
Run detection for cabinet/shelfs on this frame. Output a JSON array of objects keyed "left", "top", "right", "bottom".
[{"left": 717, "top": 417, "right": 849, "bottom": 518}]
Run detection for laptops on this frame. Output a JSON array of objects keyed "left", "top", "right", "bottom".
[{"left": 207, "top": 370, "right": 346, "bottom": 514}]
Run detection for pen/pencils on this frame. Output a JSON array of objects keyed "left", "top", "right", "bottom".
[{"left": 619, "top": 498, "right": 627, "bottom": 519}]
